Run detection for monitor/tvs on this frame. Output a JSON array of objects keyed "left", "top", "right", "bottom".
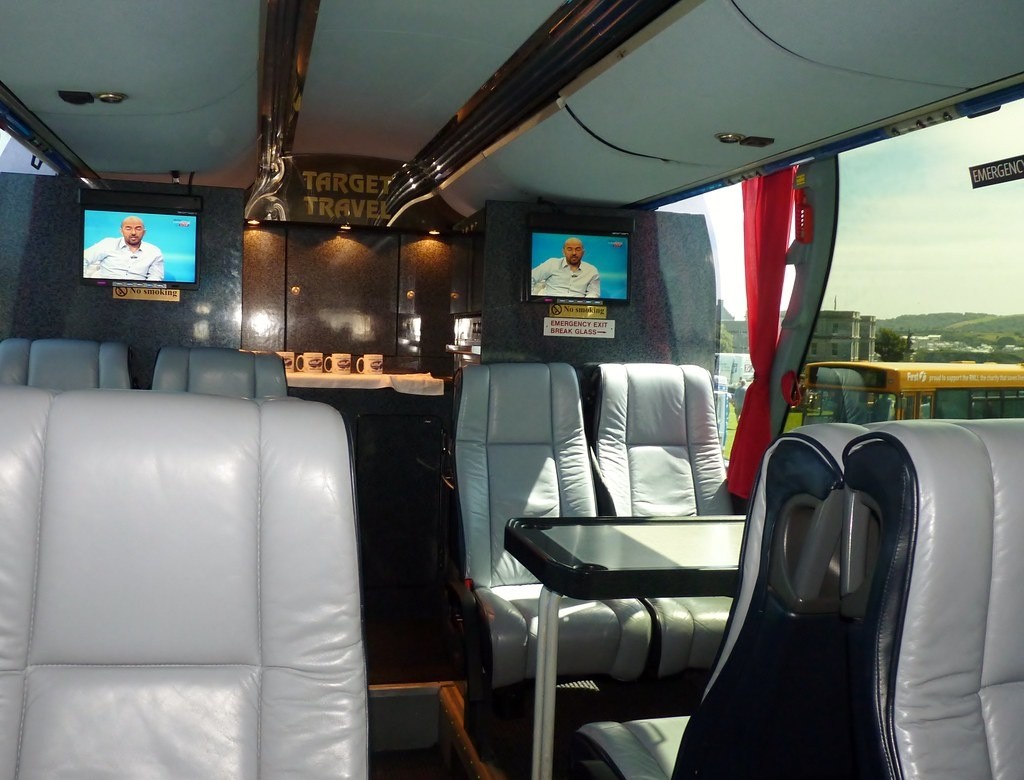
[
  {"left": 78, "top": 203, "right": 201, "bottom": 291},
  {"left": 521, "top": 228, "right": 632, "bottom": 307}
]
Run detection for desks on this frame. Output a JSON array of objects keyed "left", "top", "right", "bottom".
[
  {"left": 285, "top": 369, "right": 467, "bottom": 649},
  {"left": 503, "top": 514, "right": 749, "bottom": 780}
]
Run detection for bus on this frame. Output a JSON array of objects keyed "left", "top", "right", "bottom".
[{"left": 792, "top": 359, "right": 1024, "bottom": 427}]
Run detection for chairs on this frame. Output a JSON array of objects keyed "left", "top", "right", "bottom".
[
  {"left": 0, "top": 338, "right": 140, "bottom": 391},
  {"left": 580, "top": 363, "right": 737, "bottom": 719},
  {"left": 555, "top": 421, "right": 897, "bottom": 780},
  {"left": 0, "top": 387, "right": 372, "bottom": 779},
  {"left": 802, "top": 417, "right": 1023, "bottom": 780},
  {"left": 441, "top": 361, "right": 655, "bottom": 764},
  {"left": 151, "top": 347, "right": 288, "bottom": 398}
]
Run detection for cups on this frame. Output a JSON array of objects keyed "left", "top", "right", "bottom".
[
  {"left": 238, "top": 349, "right": 294, "bottom": 372},
  {"left": 356, "top": 354, "right": 383, "bottom": 375},
  {"left": 296, "top": 352, "right": 323, "bottom": 374},
  {"left": 324, "top": 352, "right": 351, "bottom": 374}
]
